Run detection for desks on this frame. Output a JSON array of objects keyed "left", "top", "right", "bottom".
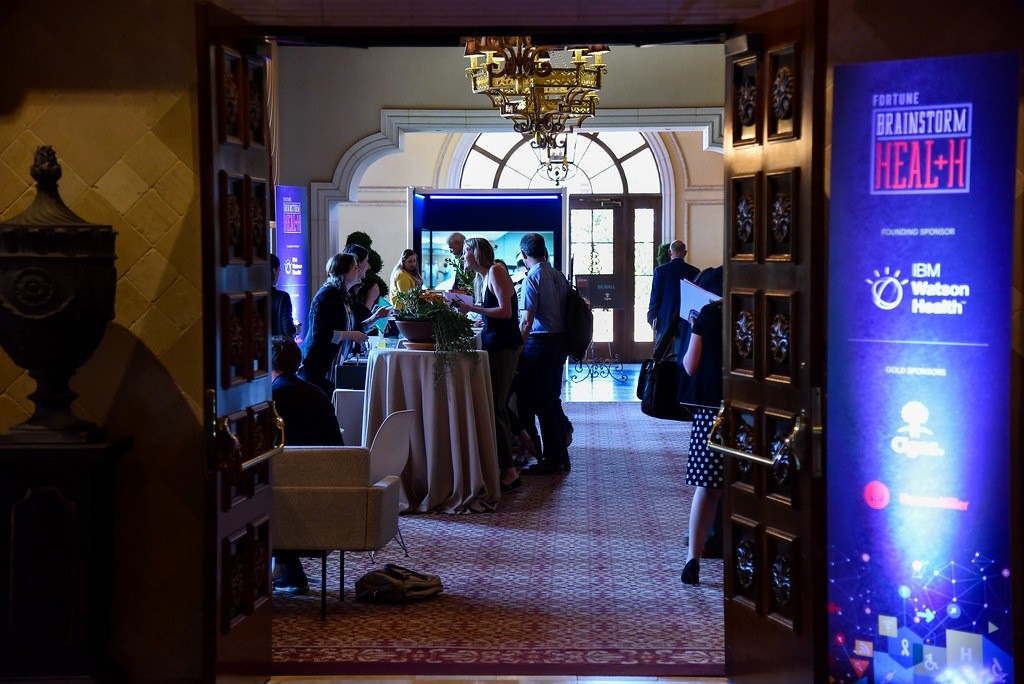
[
  {"left": 331, "top": 389, "right": 366, "bottom": 447},
  {"left": 361, "top": 347, "right": 502, "bottom": 514}
]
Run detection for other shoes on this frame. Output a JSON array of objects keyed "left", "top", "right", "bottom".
[
  {"left": 521, "top": 453, "right": 571, "bottom": 475},
  {"left": 514, "top": 457, "right": 528, "bottom": 467},
  {"left": 500, "top": 476, "right": 522, "bottom": 494},
  {"left": 272, "top": 569, "right": 310, "bottom": 592}
]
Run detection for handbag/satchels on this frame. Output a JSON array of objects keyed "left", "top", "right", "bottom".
[
  {"left": 635, "top": 352, "right": 693, "bottom": 421},
  {"left": 356, "top": 564, "right": 444, "bottom": 604}
]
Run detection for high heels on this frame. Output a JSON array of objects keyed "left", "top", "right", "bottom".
[{"left": 681, "top": 558, "right": 700, "bottom": 586}]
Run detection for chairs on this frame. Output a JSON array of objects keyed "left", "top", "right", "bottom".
[{"left": 268, "top": 409, "right": 419, "bottom": 621}]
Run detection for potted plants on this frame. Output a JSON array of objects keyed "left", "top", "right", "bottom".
[{"left": 394, "top": 285, "right": 481, "bottom": 401}]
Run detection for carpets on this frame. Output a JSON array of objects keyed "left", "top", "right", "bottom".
[{"left": 272, "top": 402, "right": 722, "bottom": 677}]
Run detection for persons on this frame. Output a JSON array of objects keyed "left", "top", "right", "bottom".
[
  {"left": 270, "top": 231, "right": 574, "bottom": 493},
  {"left": 681, "top": 298, "right": 723, "bottom": 586},
  {"left": 270, "top": 336, "right": 343, "bottom": 594},
  {"left": 647, "top": 240, "right": 723, "bottom": 557}
]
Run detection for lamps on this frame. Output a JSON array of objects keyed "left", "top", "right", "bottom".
[
  {"left": 430, "top": 195, "right": 559, "bottom": 199},
  {"left": 462, "top": 34, "right": 612, "bottom": 187}
]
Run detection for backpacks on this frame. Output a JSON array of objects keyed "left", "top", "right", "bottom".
[{"left": 554, "top": 267, "right": 594, "bottom": 361}]
